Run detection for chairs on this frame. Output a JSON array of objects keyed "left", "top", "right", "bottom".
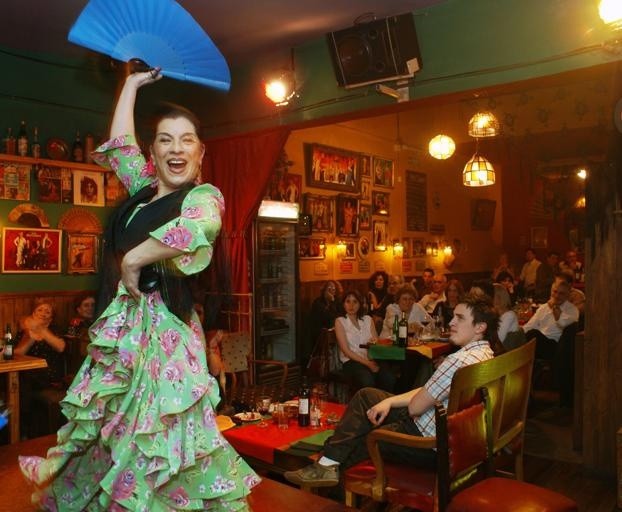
[
  {"left": 213, "top": 332, "right": 288, "bottom": 409},
  {"left": 540, "top": 322, "right": 577, "bottom": 405},
  {"left": 321, "top": 328, "right": 355, "bottom": 400},
  {"left": 433, "top": 388, "right": 579, "bottom": 511}
]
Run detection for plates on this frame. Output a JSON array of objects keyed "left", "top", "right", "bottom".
[
  {"left": 417, "top": 333, "right": 449, "bottom": 341},
  {"left": 235, "top": 412, "right": 262, "bottom": 420}
]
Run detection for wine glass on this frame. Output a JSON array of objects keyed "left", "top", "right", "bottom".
[
  {"left": 420, "top": 312, "right": 442, "bottom": 342},
  {"left": 255, "top": 396, "right": 272, "bottom": 428}
]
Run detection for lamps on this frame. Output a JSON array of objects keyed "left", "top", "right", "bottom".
[
  {"left": 392, "top": 239, "right": 405, "bottom": 261},
  {"left": 260, "top": 44, "right": 303, "bottom": 110},
  {"left": 458, "top": 95, "right": 507, "bottom": 188},
  {"left": 440, "top": 238, "right": 453, "bottom": 257}
]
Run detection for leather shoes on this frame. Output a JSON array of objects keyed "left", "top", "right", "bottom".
[{"left": 284, "top": 461, "right": 341, "bottom": 488}]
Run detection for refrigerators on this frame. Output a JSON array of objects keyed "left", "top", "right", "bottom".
[{"left": 225, "top": 172, "right": 304, "bottom": 387}]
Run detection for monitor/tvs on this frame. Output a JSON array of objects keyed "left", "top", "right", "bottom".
[
  {"left": 471, "top": 198, "right": 496, "bottom": 231},
  {"left": 325, "top": 13, "right": 423, "bottom": 90}
]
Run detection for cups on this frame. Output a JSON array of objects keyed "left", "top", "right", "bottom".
[{"left": 279, "top": 404, "right": 289, "bottom": 428}]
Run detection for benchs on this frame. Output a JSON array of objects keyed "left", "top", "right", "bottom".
[
  {"left": 369, "top": 339, "right": 536, "bottom": 511},
  {"left": 35, "top": 333, "right": 94, "bottom": 412}
]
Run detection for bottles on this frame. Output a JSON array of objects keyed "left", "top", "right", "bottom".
[
  {"left": 258, "top": 229, "right": 287, "bottom": 249},
  {"left": 298, "top": 378, "right": 310, "bottom": 426},
  {"left": 259, "top": 253, "right": 285, "bottom": 278},
  {"left": 257, "top": 331, "right": 273, "bottom": 361},
  {"left": 393, "top": 310, "right": 409, "bottom": 347},
  {"left": 256, "top": 284, "right": 284, "bottom": 305},
  {"left": 575, "top": 265, "right": 585, "bottom": 283},
  {"left": 3, "top": 322, "right": 16, "bottom": 359},
  {"left": 310, "top": 401, "right": 320, "bottom": 427},
  {"left": 0, "top": 122, "right": 96, "bottom": 164}
]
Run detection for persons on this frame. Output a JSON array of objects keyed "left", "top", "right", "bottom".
[
  {"left": 280, "top": 295, "right": 502, "bottom": 488},
  {"left": 279, "top": 150, "right": 437, "bottom": 258},
  {"left": 75, "top": 296, "right": 100, "bottom": 324},
  {"left": 18, "top": 68, "right": 265, "bottom": 512},
  {"left": 469, "top": 248, "right": 585, "bottom": 356},
  {"left": 3, "top": 162, "right": 103, "bottom": 275},
  {"left": 311, "top": 269, "right": 467, "bottom": 387},
  {"left": 12, "top": 301, "right": 69, "bottom": 442}
]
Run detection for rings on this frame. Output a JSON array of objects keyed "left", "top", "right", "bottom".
[
  {"left": 150, "top": 69, "right": 157, "bottom": 78},
  {"left": 143, "top": 72, "right": 151, "bottom": 80}
]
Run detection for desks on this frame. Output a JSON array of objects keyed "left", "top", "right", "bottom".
[{"left": 0, "top": 351, "right": 48, "bottom": 441}]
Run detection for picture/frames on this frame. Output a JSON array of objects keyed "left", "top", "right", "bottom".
[
  {"left": 1, "top": 227, "right": 62, "bottom": 276},
  {"left": 69, "top": 235, "right": 99, "bottom": 273},
  {"left": 281, "top": 142, "right": 396, "bottom": 273}
]
